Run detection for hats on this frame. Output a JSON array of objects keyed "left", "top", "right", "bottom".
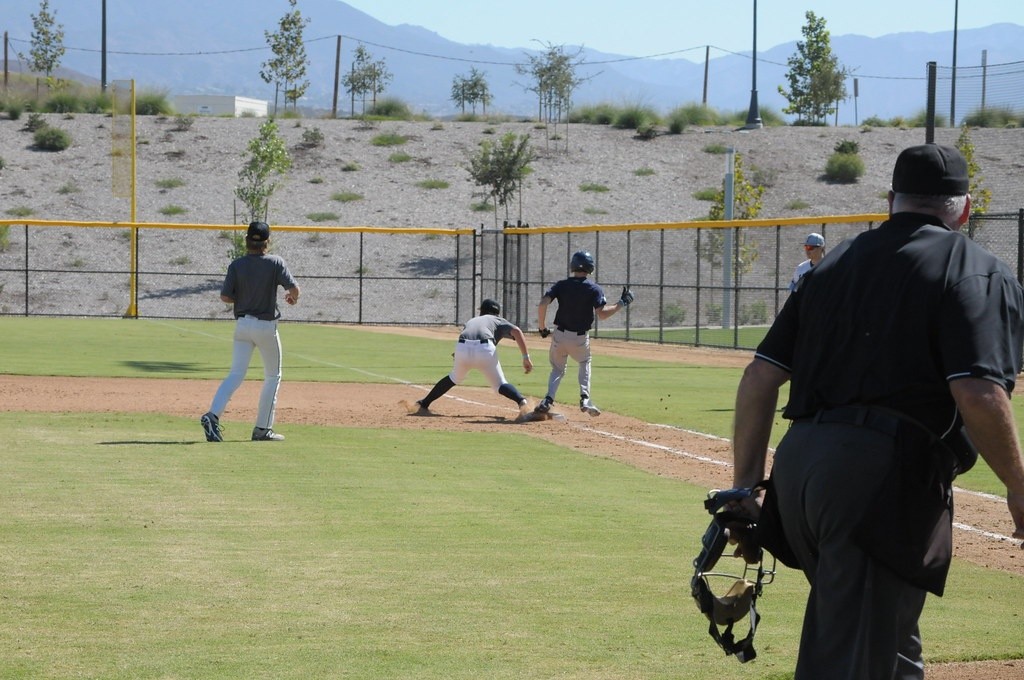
[
  {"left": 477, "top": 299, "right": 500, "bottom": 314},
  {"left": 892, "top": 142, "right": 969, "bottom": 196},
  {"left": 799, "top": 233, "right": 825, "bottom": 246},
  {"left": 246, "top": 222, "right": 269, "bottom": 241}
]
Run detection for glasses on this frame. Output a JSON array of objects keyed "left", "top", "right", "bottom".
[{"left": 805, "top": 246, "right": 821, "bottom": 250}]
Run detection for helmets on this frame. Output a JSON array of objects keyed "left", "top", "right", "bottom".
[{"left": 570, "top": 251, "right": 594, "bottom": 275}]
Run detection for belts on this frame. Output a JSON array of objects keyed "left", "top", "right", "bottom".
[
  {"left": 237, "top": 313, "right": 271, "bottom": 321},
  {"left": 459, "top": 338, "right": 488, "bottom": 344},
  {"left": 556, "top": 326, "right": 585, "bottom": 336},
  {"left": 794, "top": 407, "right": 955, "bottom": 477}
]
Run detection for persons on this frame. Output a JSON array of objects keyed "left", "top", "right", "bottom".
[
  {"left": 533, "top": 250, "right": 635, "bottom": 419},
  {"left": 417, "top": 299, "right": 533, "bottom": 415},
  {"left": 201, "top": 220, "right": 301, "bottom": 441},
  {"left": 789, "top": 232, "right": 827, "bottom": 289},
  {"left": 731, "top": 143, "right": 1024, "bottom": 680}
]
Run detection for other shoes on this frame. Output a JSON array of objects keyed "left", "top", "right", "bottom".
[
  {"left": 416, "top": 400, "right": 429, "bottom": 412},
  {"left": 518, "top": 398, "right": 527, "bottom": 409}
]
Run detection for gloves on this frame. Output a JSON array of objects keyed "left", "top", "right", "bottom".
[
  {"left": 619, "top": 284, "right": 634, "bottom": 306},
  {"left": 539, "top": 328, "right": 551, "bottom": 338}
]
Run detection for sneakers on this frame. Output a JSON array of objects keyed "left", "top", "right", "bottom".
[
  {"left": 534, "top": 398, "right": 551, "bottom": 414},
  {"left": 580, "top": 399, "right": 601, "bottom": 417},
  {"left": 251, "top": 427, "right": 285, "bottom": 441},
  {"left": 201, "top": 412, "right": 225, "bottom": 442}
]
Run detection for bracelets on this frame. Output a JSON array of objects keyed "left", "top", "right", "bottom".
[{"left": 523, "top": 354, "right": 529, "bottom": 358}]
